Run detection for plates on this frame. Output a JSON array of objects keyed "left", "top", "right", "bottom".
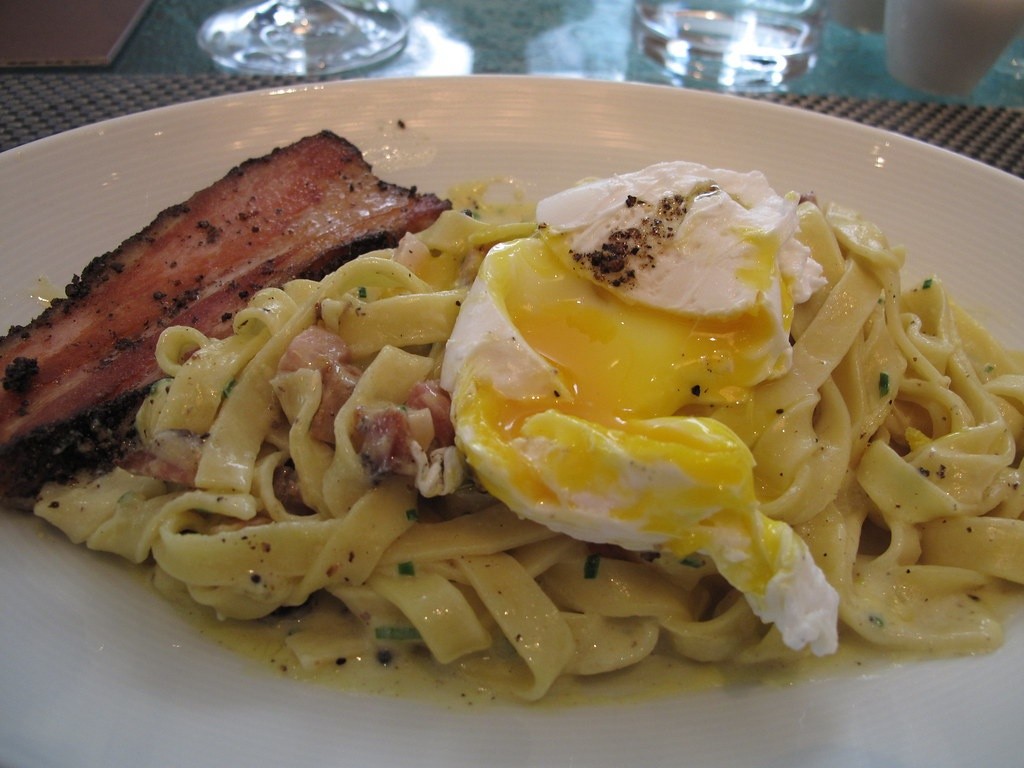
[{"left": 0, "top": 74, "right": 1024, "bottom": 768}]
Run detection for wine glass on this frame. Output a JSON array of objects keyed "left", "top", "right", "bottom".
[{"left": 196, "top": 0, "right": 411, "bottom": 77}]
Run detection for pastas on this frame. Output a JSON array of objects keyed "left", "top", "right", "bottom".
[{"left": 29, "top": 172, "right": 1024, "bottom": 705}]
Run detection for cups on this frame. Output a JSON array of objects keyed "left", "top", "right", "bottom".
[
  {"left": 878, "top": 0, "right": 1024, "bottom": 99},
  {"left": 633, "top": 0, "right": 827, "bottom": 97}
]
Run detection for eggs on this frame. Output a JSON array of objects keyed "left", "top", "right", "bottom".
[{"left": 442, "top": 158, "right": 844, "bottom": 658}]
